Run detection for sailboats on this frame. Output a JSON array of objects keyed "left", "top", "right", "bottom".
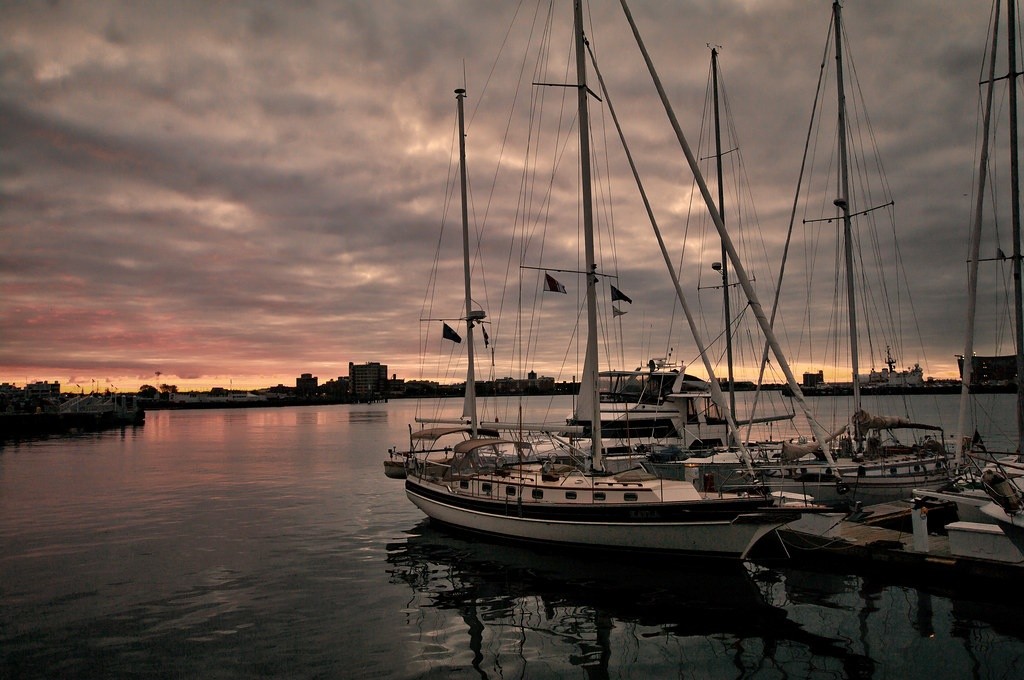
[{"left": 383, "top": 0, "right": 1024, "bottom": 566}]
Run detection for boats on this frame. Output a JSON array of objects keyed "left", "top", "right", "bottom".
[{"left": 559, "top": 358, "right": 732, "bottom": 448}]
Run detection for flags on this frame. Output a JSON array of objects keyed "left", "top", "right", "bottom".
[
  {"left": 543, "top": 273, "right": 567, "bottom": 294},
  {"left": 482, "top": 323, "right": 489, "bottom": 349},
  {"left": 613, "top": 306, "right": 628, "bottom": 318},
  {"left": 610, "top": 284, "right": 633, "bottom": 304},
  {"left": 442, "top": 323, "right": 462, "bottom": 344}
]
